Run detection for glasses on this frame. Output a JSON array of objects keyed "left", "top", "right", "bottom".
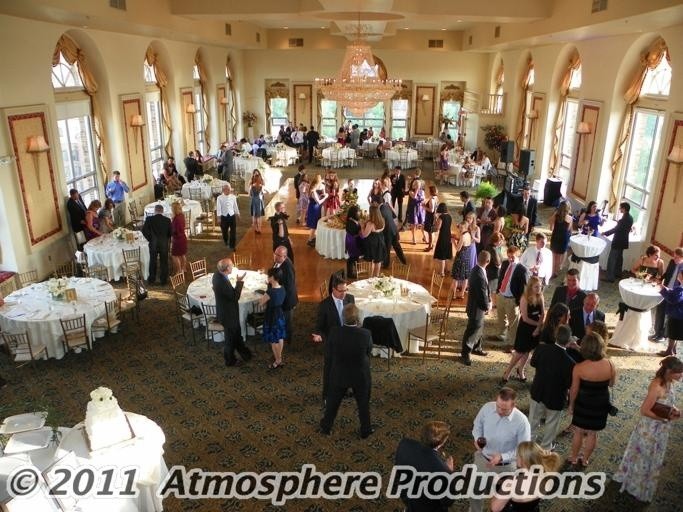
[{"left": 336, "top": 288, "right": 349, "bottom": 293}]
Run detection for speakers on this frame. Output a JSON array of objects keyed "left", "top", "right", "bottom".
[
  {"left": 519, "top": 148, "right": 535, "bottom": 175},
  {"left": 544, "top": 178, "right": 562, "bottom": 207},
  {"left": 500, "top": 140, "right": 514, "bottom": 163}
]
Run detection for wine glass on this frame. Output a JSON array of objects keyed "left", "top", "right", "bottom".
[{"left": 257, "top": 268, "right": 264, "bottom": 282}]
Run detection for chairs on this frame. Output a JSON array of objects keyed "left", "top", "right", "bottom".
[{"left": 313, "top": 257, "right": 457, "bottom": 376}]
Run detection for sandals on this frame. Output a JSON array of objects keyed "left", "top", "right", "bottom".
[
  {"left": 566, "top": 453, "right": 590, "bottom": 468},
  {"left": 267, "top": 354, "right": 285, "bottom": 370}
]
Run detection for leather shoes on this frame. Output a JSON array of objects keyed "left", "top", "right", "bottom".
[
  {"left": 462, "top": 332, "right": 516, "bottom": 366},
  {"left": 600, "top": 270, "right": 623, "bottom": 284},
  {"left": 362, "top": 423, "right": 380, "bottom": 439},
  {"left": 320, "top": 415, "right": 335, "bottom": 438},
  {"left": 647, "top": 332, "right": 668, "bottom": 343},
  {"left": 545, "top": 441, "right": 559, "bottom": 453},
  {"left": 224, "top": 351, "right": 258, "bottom": 367}
]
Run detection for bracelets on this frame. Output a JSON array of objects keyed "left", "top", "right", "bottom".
[{"left": 499, "top": 454, "right": 502, "bottom": 462}]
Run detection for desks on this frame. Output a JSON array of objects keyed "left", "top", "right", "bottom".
[
  {"left": 609, "top": 277, "right": 668, "bottom": 352},
  {"left": 565, "top": 234, "right": 608, "bottom": 294}
]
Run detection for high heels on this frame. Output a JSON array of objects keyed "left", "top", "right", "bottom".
[
  {"left": 501, "top": 367, "right": 528, "bottom": 384},
  {"left": 437, "top": 269, "right": 469, "bottom": 301},
  {"left": 656, "top": 348, "right": 678, "bottom": 360},
  {"left": 411, "top": 236, "right": 435, "bottom": 254}
]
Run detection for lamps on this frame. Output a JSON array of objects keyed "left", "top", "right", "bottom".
[
  {"left": 525, "top": 110, "right": 538, "bottom": 141},
  {"left": 297, "top": 93, "right": 306, "bottom": 117},
  {"left": 420, "top": 95, "right": 430, "bottom": 117},
  {"left": 305, "top": 6, "right": 409, "bottom": 118},
  {"left": 575, "top": 122, "right": 593, "bottom": 164},
  {"left": 665, "top": 144, "right": 682, "bottom": 205},
  {"left": 127, "top": 97, "right": 230, "bottom": 155}
]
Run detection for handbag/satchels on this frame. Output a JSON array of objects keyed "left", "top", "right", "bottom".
[{"left": 607, "top": 359, "right": 618, "bottom": 418}]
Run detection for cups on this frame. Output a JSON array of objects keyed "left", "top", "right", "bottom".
[{"left": 477, "top": 436, "right": 487, "bottom": 449}]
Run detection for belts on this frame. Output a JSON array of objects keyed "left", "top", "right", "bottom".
[
  {"left": 481, "top": 452, "right": 511, "bottom": 467},
  {"left": 503, "top": 295, "right": 515, "bottom": 300}
]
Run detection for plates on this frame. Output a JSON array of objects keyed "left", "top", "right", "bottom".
[
  {"left": 0, "top": 410, "right": 47, "bottom": 433},
  {"left": 3, "top": 427, "right": 52, "bottom": 454},
  {"left": 0, "top": 276, "right": 109, "bottom": 321}
]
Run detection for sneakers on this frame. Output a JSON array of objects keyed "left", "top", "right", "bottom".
[
  {"left": 255, "top": 230, "right": 261, "bottom": 235},
  {"left": 295, "top": 213, "right": 308, "bottom": 229},
  {"left": 306, "top": 236, "right": 316, "bottom": 249},
  {"left": 347, "top": 269, "right": 357, "bottom": 281},
  {"left": 396, "top": 216, "right": 423, "bottom": 233},
  {"left": 223, "top": 239, "right": 237, "bottom": 252},
  {"left": 147, "top": 268, "right": 188, "bottom": 287}
]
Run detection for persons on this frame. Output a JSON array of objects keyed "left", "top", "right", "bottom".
[
  {"left": 396, "top": 420, "right": 455, "bottom": 512},
  {"left": 67, "top": 120, "right": 682, "bottom": 466},
  {"left": 492, "top": 440, "right": 561, "bottom": 512},
  {"left": 469, "top": 387, "right": 532, "bottom": 511},
  {"left": 613, "top": 355, "right": 683, "bottom": 503}
]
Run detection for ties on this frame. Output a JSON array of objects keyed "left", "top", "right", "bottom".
[
  {"left": 336, "top": 299, "right": 343, "bottom": 327},
  {"left": 534, "top": 250, "right": 542, "bottom": 276},
  {"left": 500, "top": 263, "right": 515, "bottom": 293},
  {"left": 584, "top": 312, "right": 591, "bottom": 328},
  {"left": 668, "top": 264, "right": 679, "bottom": 291}
]
